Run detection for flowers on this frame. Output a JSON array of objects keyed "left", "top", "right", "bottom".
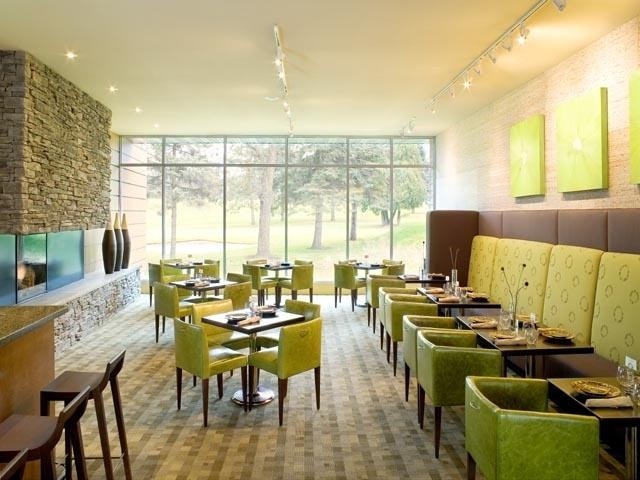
[
  {"left": 449, "top": 247, "right": 461, "bottom": 269},
  {"left": 500, "top": 263, "right": 529, "bottom": 328}
]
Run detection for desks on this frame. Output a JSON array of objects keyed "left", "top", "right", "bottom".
[
  {"left": 418, "top": 287, "right": 501, "bottom": 316},
  {"left": 546, "top": 377, "right": 638, "bottom": 479},
  {"left": 459, "top": 315, "right": 596, "bottom": 377},
  {"left": 1, "top": 307, "right": 71, "bottom": 480}
]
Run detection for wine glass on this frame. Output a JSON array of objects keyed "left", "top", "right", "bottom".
[
  {"left": 425, "top": 270, "right": 640, "bottom": 412},
  {"left": 196, "top": 273, "right": 203, "bottom": 283},
  {"left": 248, "top": 294, "right": 258, "bottom": 318}
]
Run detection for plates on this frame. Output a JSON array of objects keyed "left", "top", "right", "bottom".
[{"left": 225, "top": 313, "right": 249, "bottom": 321}]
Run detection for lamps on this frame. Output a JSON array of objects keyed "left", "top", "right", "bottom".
[{"left": 448, "top": 0, "right": 566, "bottom": 99}]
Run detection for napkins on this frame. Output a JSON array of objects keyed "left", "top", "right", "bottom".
[
  {"left": 471, "top": 321, "right": 498, "bottom": 328},
  {"left": 495, "top": 336, "right": 528, "bottom": 345},
  {"left": 586, "top": 396, "right": 634, "bottom": 408},
  {"left": 439, "top": 296, "right": 459, "bottom": 303},
  {"left": 425, "top": 288, "right": 445, "bottom": 294}
]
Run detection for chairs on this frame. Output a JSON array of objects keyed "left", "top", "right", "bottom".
[
  {"left": 465, "top": 375, "right": 599, "bottom": 480},
  {"left": 0, "top": 386, "right": 91, "bottom": 480},
  {"left": 40, "top": 349, "right": 132, "bottom": 479},
  {"left": 418, "top": 330, "right": 505, "bottom": 460},
  {"left": 336, "top": 259, "right": 460, "bottom": 402},
  {"left": 0, "top": 447, "right": 28, "bottom": 480},
  {"left": 149, "top": 259, "right": 326, "bottom": 426}
]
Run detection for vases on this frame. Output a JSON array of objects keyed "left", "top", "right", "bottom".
[
  {"left": 509, "top": 302, "right": 520, "bottom": 330},
  {"left": 451, "top": 269, "right": 458, "bottom": 294}
]
